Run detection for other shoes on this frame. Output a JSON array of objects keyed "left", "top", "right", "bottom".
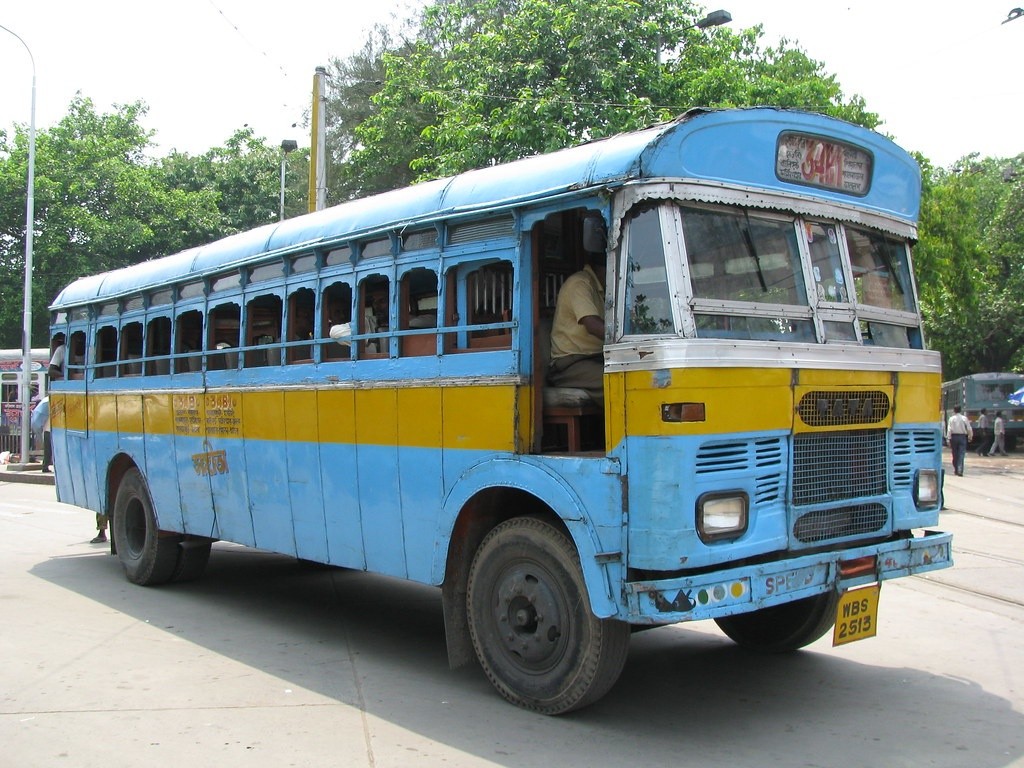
[
  {"left": 42, "top": 469, "right": 53, "bottom": 473},
  {"left": 91, "top": 536, "right": 107, "bottom": 544},
  {"left": 954, "top": 471, "right": 964, "bottom": 477},
  {"left": 973, "top": 453, "right": 1010, "bottom": 457}
]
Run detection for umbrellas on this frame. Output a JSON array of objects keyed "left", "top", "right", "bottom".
[{"left": 30, "top": 395, "right": 52, "bottom": 433}]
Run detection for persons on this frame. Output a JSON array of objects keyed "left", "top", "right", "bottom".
[
  {"left": 48, "top": 343, "right": 66, "bottom": 379},
  {"left": 277, "top": 299, "right": 313, "bottom": 366},
  {"left": 973, "top": 408, "right": 991, "bottom": 457},
  {"left": 947, "top": 404, "right": 973, "bottom": 476},
  {"left": 328, "top": 279, "right": 438, "bottom": 354},
  {"left": 986, "top": 409, "right": 1009, "bottom": 458},
  {"left": 321, "top": 297, "right": 351, "bottom": 358},
  {"left": 89, "top": 513, "right": 109, "bottom": 543},
  {"left": 545, "top": 250, "right": 607, "bottom": 411},
  {"left": 40, "top": 415, "right": 55, "bottom": 474}
]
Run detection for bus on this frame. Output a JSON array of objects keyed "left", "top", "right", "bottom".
[
  {"left": 941, "top": 371, "right": 1023, "bottom": 451},
  {"left": 53, "top": 105, "right": 952, "bottom": 716}
]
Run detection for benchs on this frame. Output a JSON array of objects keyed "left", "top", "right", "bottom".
[{"left": 51, "top": 268, "right": 606, "bottom": 455}]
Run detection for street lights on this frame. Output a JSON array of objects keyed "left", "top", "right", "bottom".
[
  {"left": 656, "top": 9, "right": 732, "bottom": 95},
  {"left": 279, "top": 140, "right": 297, "bottom": 220}
]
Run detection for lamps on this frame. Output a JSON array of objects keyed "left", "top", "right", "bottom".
[{"left": 696, "top": 10, "right": 733, "bottom": 28}]
